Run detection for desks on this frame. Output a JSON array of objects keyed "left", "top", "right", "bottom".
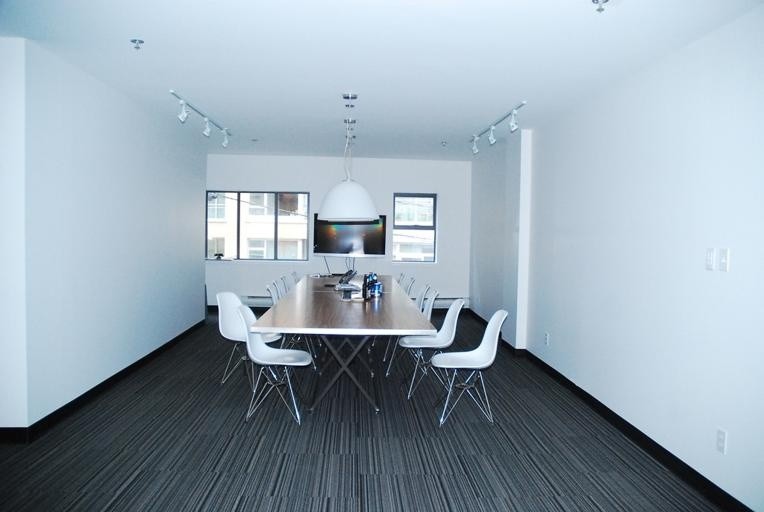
[{"left": 250, "top": 271, "right": 437, "bottom": 420}]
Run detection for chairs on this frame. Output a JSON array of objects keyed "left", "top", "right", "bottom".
[
  {"left": 404, "top": 275, "right": 415, "bottom": 296},
  {"left": 415, "top": 281, "right": 430, "bottom": 311},
  {"left": 399, "top": 298, "right": 465, "bottom": 402},
  {"left": 422, "top": 287, "right": 439, "bottom": 322},
  {"left": 239, "top": 304, "right": 312, "bottom": 428},
  {"left": 397, "top": 272, "right": 407, "bottom": 287},
  {"left": 215, "top": 291, "right": 281, "bottom": 391},
  {"left": 431, "top": 308, "right": 509, "bottom": 429},
  {"left": 265, "top": 270, "right": 299, "bottom": 306}
]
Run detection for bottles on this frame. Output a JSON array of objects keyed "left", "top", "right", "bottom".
[{"left": 362, "top": 272, "right": 377, "bottom": 300}]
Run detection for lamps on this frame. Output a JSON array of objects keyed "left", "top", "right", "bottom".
[
  {"left": 170, "top": 89, "right": 232, "bottom": 148},
  {"left": 467, "top": 100, "right": 528, "bottom": 155},
  {"left": 316, "top": 93, "right": 381, "bottom": 224}
]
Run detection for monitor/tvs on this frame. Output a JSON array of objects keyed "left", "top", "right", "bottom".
[{"left": 313, "top": 211, "right": 388, "bottom": 258}]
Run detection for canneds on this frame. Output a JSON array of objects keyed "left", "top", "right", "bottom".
[
  {"left": 373, "top": 282, "right": 383, "bottom": 297},
  {"left": 372, "top": 297, "right": 381, "bottom": 311}
]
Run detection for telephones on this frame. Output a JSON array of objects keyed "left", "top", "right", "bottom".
[{"left": 339, "top": 270, "right": 356, "bottom": 284}]
